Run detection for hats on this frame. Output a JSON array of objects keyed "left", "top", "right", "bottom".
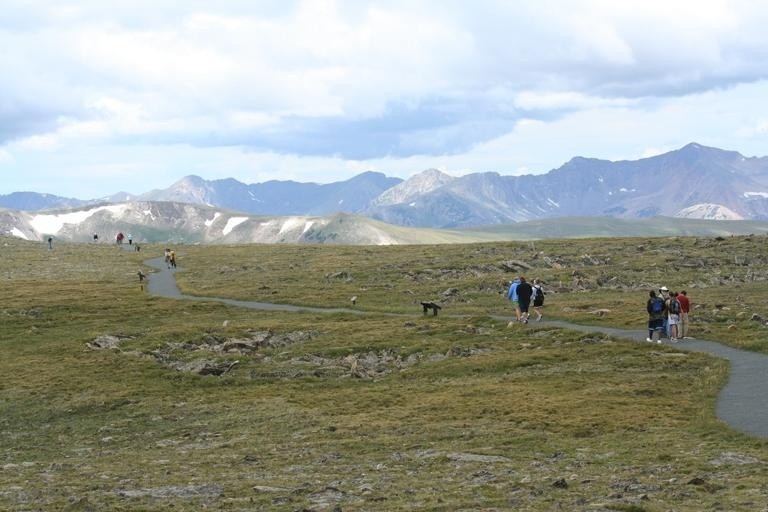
[{"left": 660, "top": 287, "right": 669, "bottom": 291}]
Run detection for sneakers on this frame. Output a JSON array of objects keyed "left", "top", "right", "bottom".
[
  {"left": 516, "top": 313, "right": 542, "bottom": 323},
  {"left": 646, "top": 337, "right": 688, "bottom": 344}
]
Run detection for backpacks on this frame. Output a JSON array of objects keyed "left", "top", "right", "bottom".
[
  {"left": 670, "top": 300, "right": 679, "bottom": 314},
  {"left": 533, "top": 286, "right": 544, "bottom": 306}
]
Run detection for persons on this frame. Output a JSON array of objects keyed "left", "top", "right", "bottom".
[
  {"left": 165, "top": 248, "right": 176, "bottom": 269},
  {"left": 647, "top": 286, "right": 689, "bottom": 343},
  {"left": 93, "top": 233, "right": 98, "bottom": 245},
  {"left": 48, "top": 236, "right": 53, "bottom": 249},
  {"left": 508, "top": 276, "right": 544, "bottom": 324},
  {"left": 115, "top": 231, "right": 140, "bottom": 252}
]
